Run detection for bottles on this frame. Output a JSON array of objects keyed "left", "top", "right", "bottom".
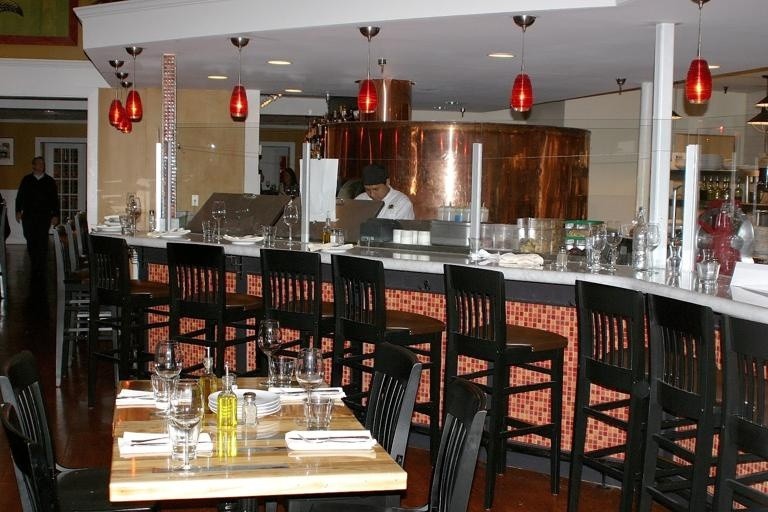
[
  {"left": 630, "top": 206, "right": 646, "bottom": 272},
  {"left": 321, "top": 218, "right": 344, "bottom": 245},
  {"left": 696, "top": 282, "right": 718, "bottom": 297},
  {"left": 699, "top": 176, "right": 740, "bottom": 202},
  {"left": 664, "top": 244, "right": 681, "bottom": 273},
  {"left": 198, "top": 358, "right": 218, "bottom": 415},
  {"left": 556, "top": 245, "right": 568, "bottom": 268},
  {"left": 694, "top": 249, "right": 720, "bottom": 285},
  {"left": 148, "top": 209, "right": 155, "bottom": 232},
  {"left": 242, "top": 392, "right": 257, "bottom": 426},
  {"left": 666, "top": 276, "right": 680, "bottom": 288},
  {"left": 216, "top": 376, "right": 237, "bottom": 432},
  {"left": 437, "top": 199, "right": 489, "bottom": 222}
]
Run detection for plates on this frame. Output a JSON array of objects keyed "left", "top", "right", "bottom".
[
  {"left": 156, "top": 229, "right": 191, "bottom": 238},
  {"left": 321, "top": 244, "right": 353, "bottom": 253},
  {"left": 96, "top": 225, "right": 121, "bottom": 232},
  {"left": 701, "top": 153, "right": 723, "bottom": 171},
  {"left": 207, "top": 389, "right": 281, "bottom": 419},
  {"left": 221, "top": 234, "right": 264, "bottom": 245},
  {"left": 752, "top": 224, "right": 767, "bottom": 257}
]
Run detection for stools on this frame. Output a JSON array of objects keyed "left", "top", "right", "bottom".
[{"left": 0, "top": 212, "right": 767, "bottom": 511}]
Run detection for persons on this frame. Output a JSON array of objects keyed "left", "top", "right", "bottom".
[
  {"left": 355, "top": 164, "right": 415, "bottom": 221},
  {"left": 281, "top": 167, "right": 296, "bottom": 198},
  {"left": 16, "top": 156, "right": 60, "bottom": 278}
]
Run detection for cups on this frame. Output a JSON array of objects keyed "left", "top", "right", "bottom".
[
  {"left": 262, "top": 225, "right": 276, "bottom": 248},
  {"left": 302, "top": 396, "right": 332, "bottom": 431},
  {"left": 168, "top": 418, "right": 198, "bottom": 461},
  {"left": 392, "top": 228, "right": 430, "bottom": 246},
  {"left": 268, "top": 356, "right": 295, "bottom": 386},
  {"left": 151, "top": 374, "right": 178, "bottom": 400},
  {"left": 120, "top": 216, "right": 134, "bottom": 235},
  {"left": 201, "top": 220, "right": 215, "bottom": 237},
  {"left": 392, "top": 253, "right": 430, "bottom": 261}
]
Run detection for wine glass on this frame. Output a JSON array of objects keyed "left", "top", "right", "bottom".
[
  {"left": 265, "top": 180, "right": 269, "bottom": 190},
  {"left": 284, "top": 204, "right": 299, "bottom": 244},
  {"left": 644, "top": 223, "right": 662, "bottom": 276},
  {"left": 586, "top": 220, "right": 622, "bottom": 273},
  {"left": 168, "top": 380, "right": 202, "bottom": 474},
  {"left": 153, "top": 340, "right": 183, "bottom": 402},
  {"left": 210, "top": 200, "right": 226, "bottom": 239},
  {"left": 257, "top": 318, "right": 283, "bottom": 386},
  {"left": 127, "top": 196, "right": 141, "bottom": 232}
]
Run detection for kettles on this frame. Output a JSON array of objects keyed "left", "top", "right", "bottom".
[{"left": 294, "top": 348, "right": 325, "bottom": 404}]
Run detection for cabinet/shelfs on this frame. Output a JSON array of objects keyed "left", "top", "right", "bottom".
[{"left": 669, "top": 167, "right": 768, "bottom": 212}]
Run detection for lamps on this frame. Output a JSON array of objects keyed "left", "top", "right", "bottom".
[
  {"left": 685, "top": 0, "right": 712, "bottom": 105},
  {"left": 230, "top": 37, "right": 249, "bottom": 120},
  {"left": 108, "top": 47, "right": 145, "bottom": 134},
  {"left": 510, "top": 15, "right": 537, "bottom": 113},
  {"left": 746, "top": 74, "right": 768, "bottom": 125},
  {"left": 358, "top": 26, "right": 380, "bottom": 114},
  {"left": 672, "top": 110, "right": 682, "bottom": 120}
]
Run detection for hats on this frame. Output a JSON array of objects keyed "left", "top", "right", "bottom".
[{"left": 362, "top": 166, "right": 387, "bottom": 185}]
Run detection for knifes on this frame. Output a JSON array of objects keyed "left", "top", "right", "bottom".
[
  {"left": 289, "top": 435, "right": 369, "bottom": 443},
  {"left": 275, "top": 389, "right": 339, "bottom": 395}
]
